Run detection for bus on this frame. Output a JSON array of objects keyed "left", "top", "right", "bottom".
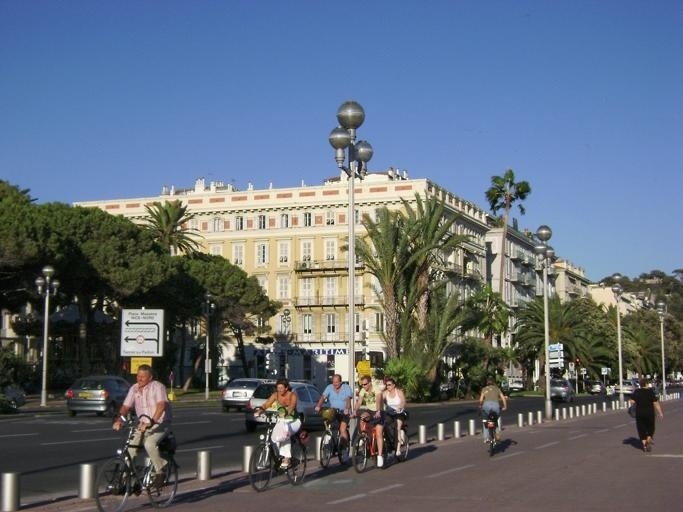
[{"left": 223, "top": 376, "right": 327, "bottom": 434}]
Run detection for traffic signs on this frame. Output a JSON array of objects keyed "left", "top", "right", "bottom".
[
  {"left": 121, "top": 309, "right": 164, "bottom": 357},
  {"left": 548, "top": 344, "right": 564, "bottom": 368}
]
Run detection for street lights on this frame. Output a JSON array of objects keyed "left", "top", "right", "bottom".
[
  {"left": 611, "top": 274, "right": 624, "bottom": 407},
  {"left": 534, "top": 222, "right": 556, "bottom": 419},
  {"left": 328, "top": 100, "right": 372, "bottom": 421},
  {"left": 35, "top": 263, "right": 61, "bottom": 407},
  {"left": 200, "top": 290, "right": 219, "bottom": 398},
  {"left": 279, "top": 309, "right": 293, "bottom": 380},
  {"left": 658, "top": 301, "right": 669, "bottom": 394}
]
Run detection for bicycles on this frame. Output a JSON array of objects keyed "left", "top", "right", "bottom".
[
  {"left": 483, "top": 414, "right": 499, "bottom": 456},
  {"left": 248, "top": 407, "right": 311, "bottom": 492},
  {"left": 318, "top": 407, "right": 410, "bottom": 472},
  {"left": 94, "top": 414, "right": 181, "bottom": 512}
]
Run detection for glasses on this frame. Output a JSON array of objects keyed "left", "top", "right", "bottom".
[
  {"left": 362, "top": 383, "right": 370, "bottom": 386},
  {"left": 386, "top": 383, "right": 392, "bottom": 386}
]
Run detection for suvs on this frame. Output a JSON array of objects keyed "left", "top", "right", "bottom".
[{"left": 550, "top": 379, "right": 575, "bottom": 403}]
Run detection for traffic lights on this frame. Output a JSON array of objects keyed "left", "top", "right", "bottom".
[{"left": 576, "top": 356, "right": 580, "bottom": 367}]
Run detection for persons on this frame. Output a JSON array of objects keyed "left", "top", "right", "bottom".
[
  {"left": 629, "top": 376, "right": 666, "bottom": 453},
  {"left": 380, "top": 378, "right": 407, "bottom": 455},
  {"left": 252, "top": 378, "right": 303, "bottom": 473},
  {"left": 311, "top": 373, "right": 353, "bottom": 451},
  {"left": 475, "top": 375, "right": 509, "bottom": 443},
  {"left": 346, "top": 374, "right": 385, "bottom": 468},
  {"left": 111, "top": 364, "right": 173, "bottom": 489}
]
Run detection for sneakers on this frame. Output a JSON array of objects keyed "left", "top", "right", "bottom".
[
  {"left": 376, "top": 454, "right": 385, "bottom": 468},
  {"left": 280, "top": 458, "right": 292, "bottom": 469}
]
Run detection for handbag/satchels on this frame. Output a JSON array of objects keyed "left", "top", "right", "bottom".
[{"left": 628, "top": 404, "right": 636, "bottom": 419}]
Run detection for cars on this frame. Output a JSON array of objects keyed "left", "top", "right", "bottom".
[
  {"left": 66, "top": 373, "right": 136, "bottom": 419},
  {"left": 586, "top": 378, "right": 683, "bottom": 397},
  {"left": 0, "top": 384, "right": 27, "bottom": 414}
]
